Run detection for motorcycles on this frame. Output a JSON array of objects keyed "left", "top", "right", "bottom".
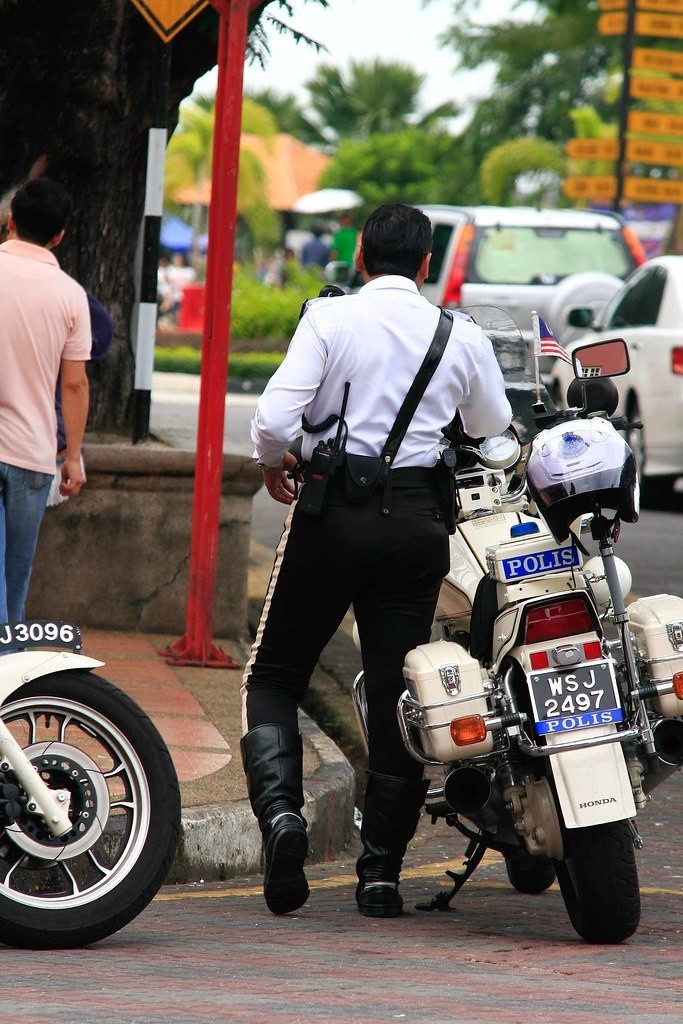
[
  {"left": 0, "top": 621, "right": 181, "bottom": 947},
  {"left": 347, "top": 303, "right": 683, "bottom": 947}
]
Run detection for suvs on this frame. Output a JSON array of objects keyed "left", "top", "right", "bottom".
[{"left": 324, "top": 202, "right": 650, "bottom": 470}]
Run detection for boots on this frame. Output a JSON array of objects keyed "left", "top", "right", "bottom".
[
  {"left": 355, "top": 770, "right": 432, "bottom": 917},
  {"left": 239, "top": 723, "right": 310, "bottom": 915}
]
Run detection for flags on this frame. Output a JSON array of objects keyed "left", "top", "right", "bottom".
[{"left": 534, "top": 316, "right": 574, "bottom": 366}]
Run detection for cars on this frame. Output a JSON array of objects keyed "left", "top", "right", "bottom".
[{"left": 542, "top": 255, "right": 683, "bottom": 489}]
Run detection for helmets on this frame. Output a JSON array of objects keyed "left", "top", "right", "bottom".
[{"left": 525, "top": 417, "right": 640, "bottom": 545}]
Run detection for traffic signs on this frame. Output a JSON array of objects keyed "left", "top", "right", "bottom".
[{"left": 562, "top": 1, "right": 683, "bottom": 208}]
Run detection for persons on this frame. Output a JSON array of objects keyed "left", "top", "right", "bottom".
[
  {"left": 156, "top": 251, "right": 195, "bottom": 317},
  {"left": 0, "top": 178, "right": 93, "bottom": 658},
  {"left": 241, "top": 204, "right": 512, "bottom": 921},
  {"left": 266, "top": 210, "right": 362, "bottom": 286}
]
